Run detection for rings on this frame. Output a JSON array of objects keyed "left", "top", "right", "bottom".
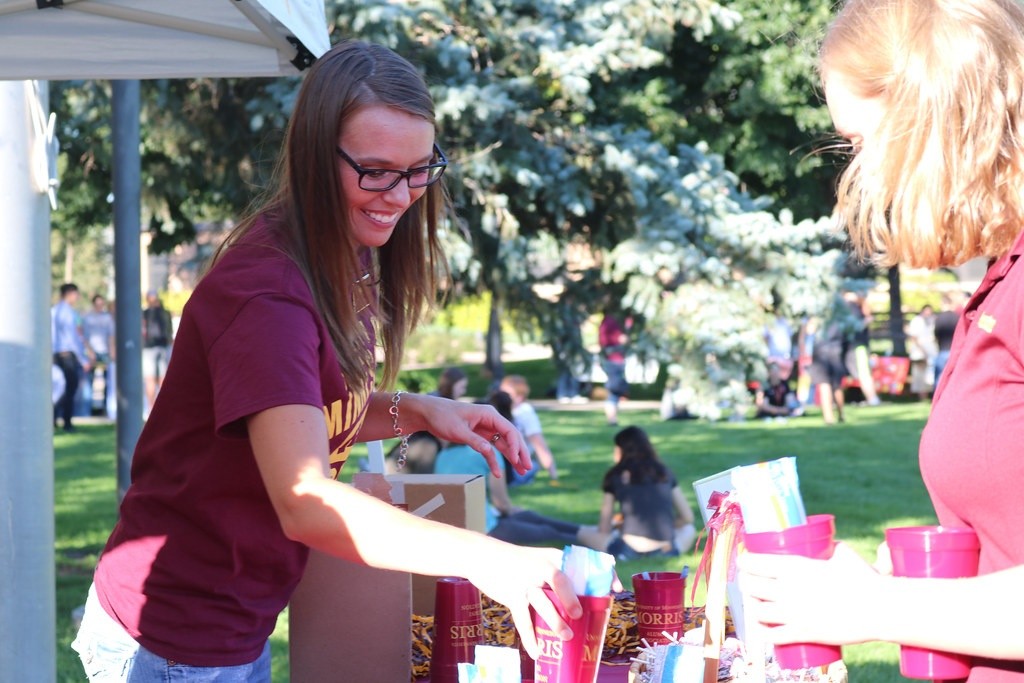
[{"left": 491, "top": 433, "right": 501, "bottom": 441}]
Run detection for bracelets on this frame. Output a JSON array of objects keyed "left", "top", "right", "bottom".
[{"left": 389, "top": 389, "right": 415, "bottom": 467}]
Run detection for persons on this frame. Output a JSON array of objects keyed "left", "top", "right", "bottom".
[
  {"left": 736, "top": 0, "right": 1024, "bottom": 683},
  {"left": 72, "top": 39, "right": 589, "bottom": 683},
  {"left": 48, "top": 283, "right": 175, "bottom": 433},
  {"left": 378, "top": 256, "right": 950, "bottom": 570}
]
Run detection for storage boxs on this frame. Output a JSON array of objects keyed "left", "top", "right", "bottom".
[{"left": 350, "top": 473, "right": 485, "bottom": 616}]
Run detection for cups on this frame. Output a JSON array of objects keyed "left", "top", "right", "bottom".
[
  {"left": 743, "top": 513, "right": 843, "bottom": 670},
  {"left": 531, "top": 589, "right": 615, "bottom": 683},
  {"left": 427, "top": 577, "right": 484, "bottom": 683},
  {"left": 513, "top": 602, "right": 541, "bottom": 680},
  {"left": 883, "top": 523, "right": 981, "bottom": 681},
  {"left": 632, "top": 571, "right": 687, "bottom": 648}
]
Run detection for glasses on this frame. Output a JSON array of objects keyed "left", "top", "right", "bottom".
[{"left": 337, "top": 144, "right": 449, "bottom": 192}]
